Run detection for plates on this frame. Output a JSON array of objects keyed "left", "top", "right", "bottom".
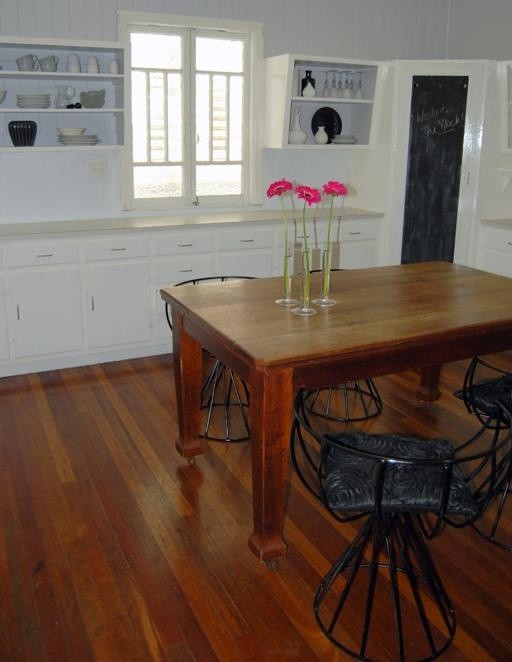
[
  {"left": 328, "top": 133, "right": 359, "bottom": 144},
  {"left": 15, "top": 94, "right": 51, "bottom": 110},
  {"left": 56, "top": 128, "right": 101, "bottom": 146},
  {"left": 309, "top": 105, "right": 343, "bottom": 144}
]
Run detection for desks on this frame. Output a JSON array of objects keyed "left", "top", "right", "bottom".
[{"left": 160, "top": 259, "right": 511, "bottom": 562}]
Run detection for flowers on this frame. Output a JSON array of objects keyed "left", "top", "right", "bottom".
[{"left": 266, "top": 178, "right": 348, "bottom": 305}]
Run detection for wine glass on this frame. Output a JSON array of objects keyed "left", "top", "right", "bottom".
[{"left": 320, "top": 69, "right": 364, "bottom": 99}]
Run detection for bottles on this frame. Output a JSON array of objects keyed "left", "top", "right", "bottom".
[
  {"left": 314, "top": 127, "right": 329, "bottom": 145},
  {"left": 300, "top": 70, "right": 316, "bottom": 98},
  {"left": 288, "top": 107, "right": 306, "bottom": 146}
]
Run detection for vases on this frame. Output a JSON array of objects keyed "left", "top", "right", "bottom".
[{"left": 275, "top": 241, "right": 338, "bottom": 317}]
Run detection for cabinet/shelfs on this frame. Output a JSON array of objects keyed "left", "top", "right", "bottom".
[
  {"left": 0, "top": 35, "right": 132, "bottom": 152},
  {"left": 149, "top": 227, "right": 268, "bottom": 336},
  {"left": 273, "top": 222, "right": 378, "bottom": 277},
  {"left": 262, "top": 53, "right": 383, "bottom": 150},
  {"left": 6, "top": 237, "right": 151, "bottom": 360}
]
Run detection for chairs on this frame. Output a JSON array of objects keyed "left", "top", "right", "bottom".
[
  {"left": 454, "top": 354, "right": 511, "bottom": 553},
  {"left": 164, "top": 276, "right": 259, "bottom": 440},
  {"left": 298, "top": 271, "right": 382, "bottom": 422},
  {"left": 311, "top": 418, "right": 508, "bottom": 661}
]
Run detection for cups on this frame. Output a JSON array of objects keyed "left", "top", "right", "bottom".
[
  {"left": 109, "top": 60, "right": 120, "bottom": 74},
  {"left": 15, "top": 53, "right": 58, "bottom": 73},
  {"left": 67, "top": 55, "right": 81, "bottom": 74},
  {"left": 86, "top": 56, "right": 99, "bottom": 73}
]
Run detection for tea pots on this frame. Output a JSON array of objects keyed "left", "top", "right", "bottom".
[{"left": 53, "top": 85, "right": 75, "bottom": 109}]
